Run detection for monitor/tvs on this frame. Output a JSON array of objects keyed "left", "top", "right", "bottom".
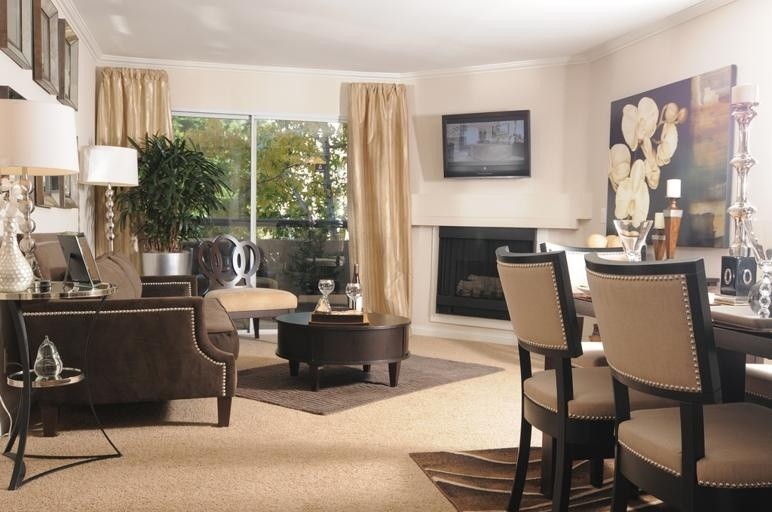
[{"left": 441, "top": 110, "right": 531, "bottom": 178}]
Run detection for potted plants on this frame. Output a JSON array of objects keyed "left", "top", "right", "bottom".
[{"left": 112, "top": 129, "right": 236, "bottom": 274}]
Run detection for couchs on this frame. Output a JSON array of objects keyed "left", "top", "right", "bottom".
[{"left": 1, "top": 229, "right": 242, "bottom": 439}]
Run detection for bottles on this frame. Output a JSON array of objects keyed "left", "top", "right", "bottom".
[{"left": 349, "top": 262, "right": 363, "bottom": 310}]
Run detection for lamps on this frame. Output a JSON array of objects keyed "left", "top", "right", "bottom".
[
  {"left": 77, "top": 143, "right": 141, "bottom": 253},
  {"left": 1, "top": 99, "right": 81, "bottom": 294}
]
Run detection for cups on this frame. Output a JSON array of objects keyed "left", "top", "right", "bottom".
[{"left": 613, "top": 219, "right": 654, "bottom": 260}]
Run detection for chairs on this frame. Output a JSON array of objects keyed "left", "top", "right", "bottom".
[
  {"left": 491, "top": 239, "right": 703, "bottom": 511},
  {"left": 579, "top": 245, "right": 771, "bottom": 511},
  {"left": 256, "top": 236, "right": 350, "bottom": 294},
  {"left": 540, "top": 241, "right": 632, "bottom": 489},
  {"left": 195, "top": 232, "right": 299, "bottom": 340}
]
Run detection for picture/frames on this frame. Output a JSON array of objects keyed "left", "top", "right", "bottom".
[
  {"left": 1, "top": 0, "right": 81, "bottom": 210},
  {"left": 55, "top": 232, "right": 102, "bottom": 289}
]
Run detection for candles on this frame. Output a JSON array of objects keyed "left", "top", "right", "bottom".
[
  {"left": 665, "top": 177, "right": 683, "bottom": 198},
  {"left": 729, "top": 83, "right": 759, "bottom": 106},
  {"left": 653, "top": 210, "right": 666, "bottom": 230}
]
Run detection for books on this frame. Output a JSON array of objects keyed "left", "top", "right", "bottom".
[{"left": 311, "top": 309, "right": 363, "bottom": 321}]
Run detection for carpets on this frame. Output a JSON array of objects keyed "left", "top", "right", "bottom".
[
  {"left": 234, "top": 352, "right": 507, "bottom": 417},
  {"left": 408, "top": 444, "right": 661, "bottom": 511}
]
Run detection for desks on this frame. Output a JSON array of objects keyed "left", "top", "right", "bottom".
[{"left": 1, "top": 278, "right": 126, "bottom": 491}]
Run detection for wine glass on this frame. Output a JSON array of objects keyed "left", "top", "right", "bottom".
[
  {"left": 318, "top": 279, "right": 335, "bottom": 312},
  {"left": 346, "top": 283, "right": 363, "bottom": 313}
]
